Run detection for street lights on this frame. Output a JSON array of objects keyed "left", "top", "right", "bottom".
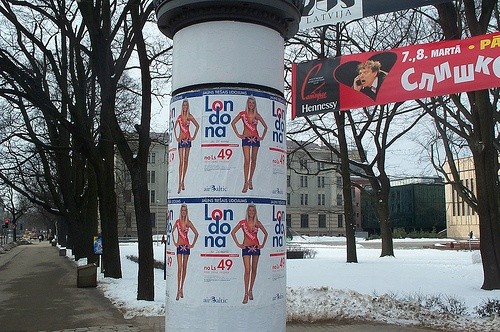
[{"left": 155, "top": 200, "right": 159, "bottom": 247}]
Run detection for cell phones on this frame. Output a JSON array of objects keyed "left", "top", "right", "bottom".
[{"left": 357, "top": 80, "right": 362, "bottom": 86}]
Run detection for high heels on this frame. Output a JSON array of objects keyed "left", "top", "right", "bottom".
[
  {"left": 176, "top": 289, "right": 184, "bottom": 300},
  {"left": 178, "top": 183, "right": 185, "bottom": 194},
  {"left": 242, "top": 180, "right": 253, "bottom": 193},
  {"left": 243, "top": 289, "right": 253, "bottom": 303}
]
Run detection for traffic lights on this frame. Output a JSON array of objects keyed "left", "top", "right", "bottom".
[{"left": 1, "top": 220, "right": 8, "bottom": 229}]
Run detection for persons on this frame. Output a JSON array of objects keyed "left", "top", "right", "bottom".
[
  {"left": 351, "top": 60, "right": 388, "bottom": 102},
  {"left": 232, "top": 203, "right": 268, "bottom": 304},
  {"left": 470, "top": 231, "right": 473, "bottom": 238},
  {"left": 171, "top": 204, "right": 199, "bottom": 300},
  {"left": 231, "top": 96, "right": 268, "bottom": 194},
  {"left": 174, "top": 99, "right": 200, "bottom": 195},
  {"left": 38, "top": 231, "right": 54, "bottom": 244}
]
